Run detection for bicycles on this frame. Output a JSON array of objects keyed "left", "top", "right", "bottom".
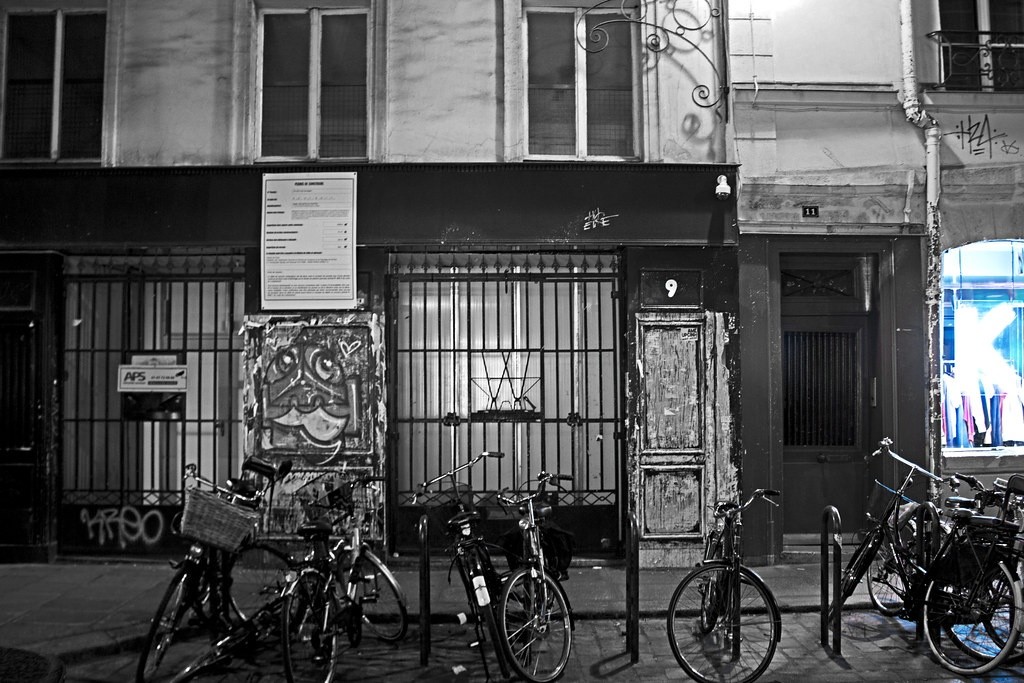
[
  {"left": 667, "top": 488, "right": 782, "bottom": 683},
  {"left": 138, "top": 463, "right": 409, "bottom": 683},
  {"left": 406, "top": 451, "right": 576, "bottom": 683},
  {"left": 828, "top": 436, "right": 1024, "bottom": 677}
]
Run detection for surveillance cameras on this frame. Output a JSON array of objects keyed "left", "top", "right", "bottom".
[{"left": 715, "top": 185, "right": 731, "bottom": 201}]
